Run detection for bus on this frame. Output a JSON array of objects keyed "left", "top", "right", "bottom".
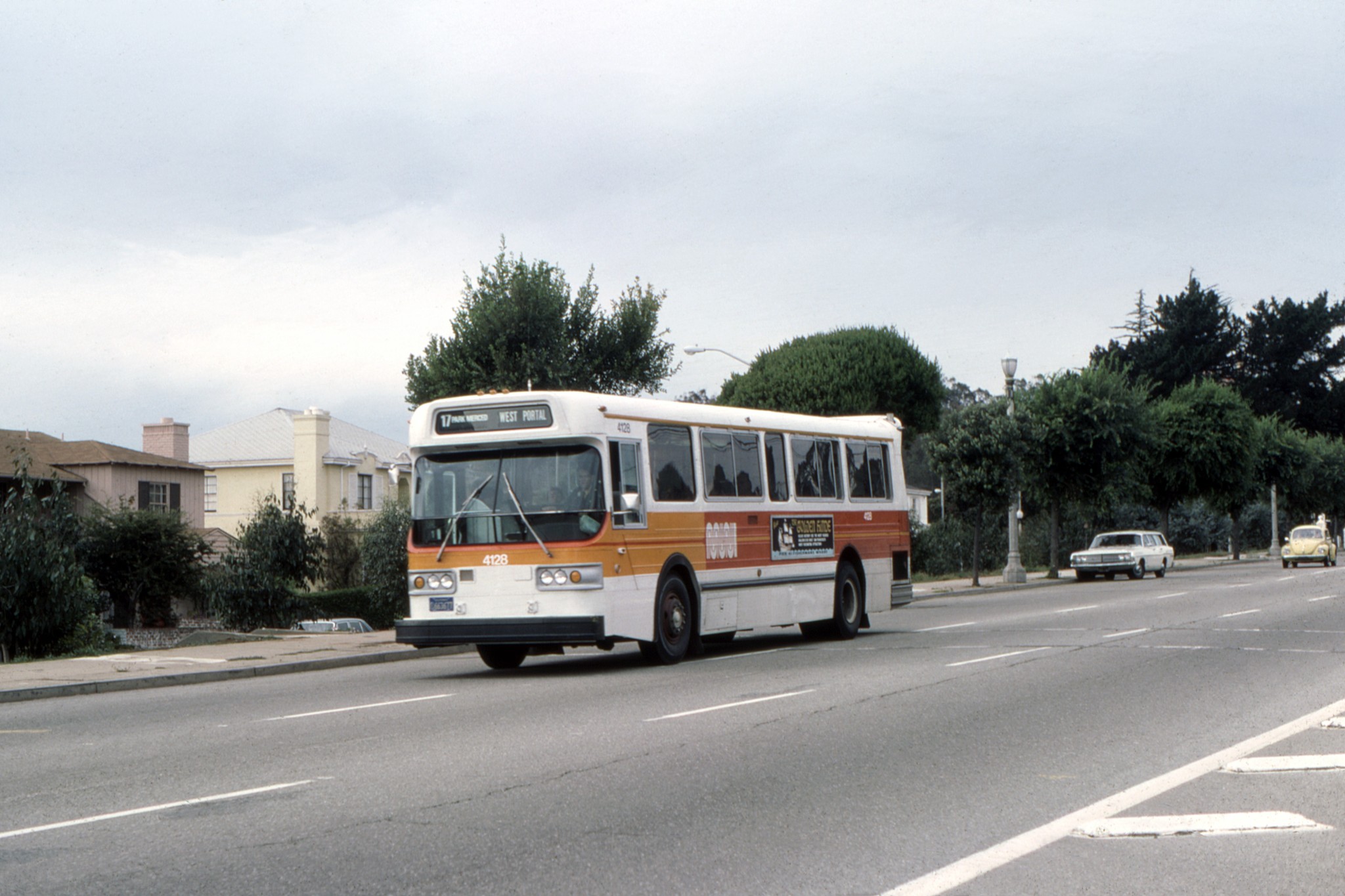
[{"left": 386, "top": 379, "right": 916, "bottom": 668}]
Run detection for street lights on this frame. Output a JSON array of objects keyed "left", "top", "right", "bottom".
[
  {"left": 683, "top": 347, "right": 780, "bottom": 411},
  {"left": 933, "top": 477, "right": 945, "bottom": 523},
  {"left": 1001, "top": 358, "right": 1027, "bottom": 583}
]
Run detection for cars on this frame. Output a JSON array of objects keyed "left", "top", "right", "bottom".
[
  {"left": 1069, "top": 530, "right": 1174, "bottom": 581},
  {"left": 1280, "top": 524, "right": 1338, "bottom": 569},
  {"left": 290, "top": 618, "right": 374, "bottom": 634}
]
